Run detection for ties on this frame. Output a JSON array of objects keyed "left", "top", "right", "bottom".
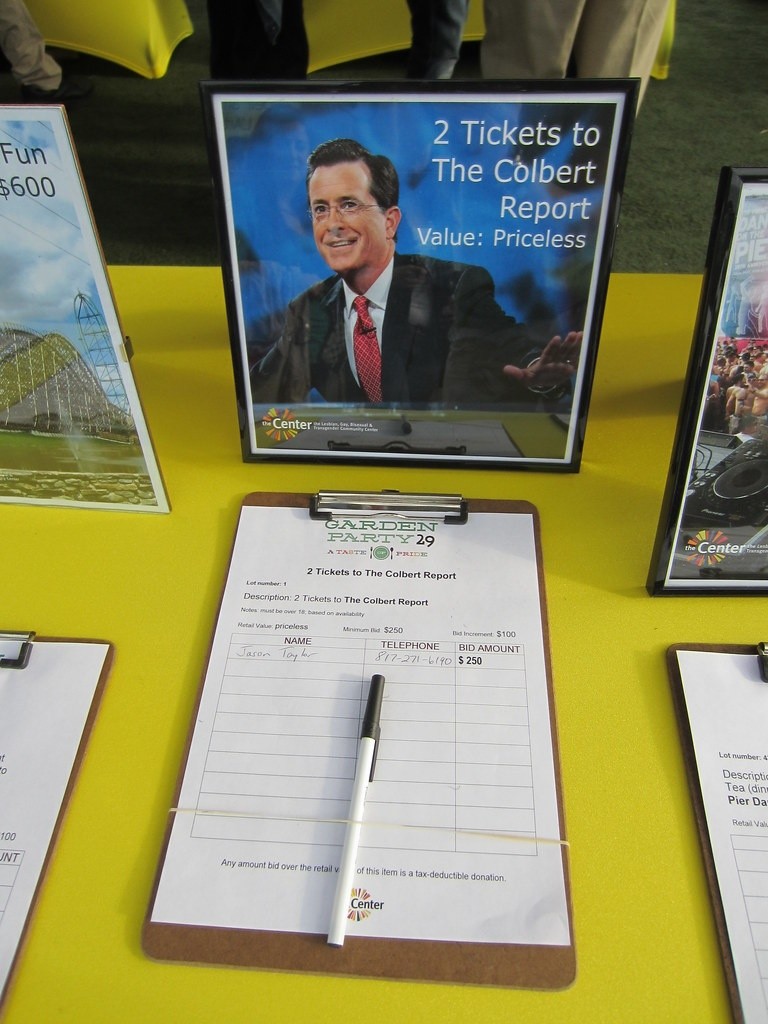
[{"left": 353, "top": 296, "right": 383, "bottom": 409}]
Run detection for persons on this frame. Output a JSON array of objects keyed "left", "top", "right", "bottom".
[
  {"left": 276, "top": 137, "right": 584, "bottom": 413},
  {"left": 405, "top": 0, "right": 470, "bottom": 82},
  {"left": 0, "top": 1, "right": 96, "bottom": 103},
  {"left": 480, "top": 0, "right": 670, "bottom": 127},
  {"left": 207, "top": 0, "right": 309, "bottom": 82},
  {"left": 702, "top": 337, "right": 768, "bottom": 444}
]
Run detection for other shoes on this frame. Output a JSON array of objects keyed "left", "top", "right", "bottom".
[{"left": 21, "top": 72, "right": 93, "bottom": 103}]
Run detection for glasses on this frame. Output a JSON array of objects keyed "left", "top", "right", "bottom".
[
  {"left": 306, "top": 200, "right": 380, "bottom": 222},
  {"left": 757, "top": 379, "right": 766, "bottom": 381}
]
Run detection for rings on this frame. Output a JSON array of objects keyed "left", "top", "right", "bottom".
[{"left": 566, "top": 358, "right": 577, "bottom": 364}]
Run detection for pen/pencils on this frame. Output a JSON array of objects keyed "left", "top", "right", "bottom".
[{"left": 324, "top": 672, "right": 385, "bottom": 946}]
